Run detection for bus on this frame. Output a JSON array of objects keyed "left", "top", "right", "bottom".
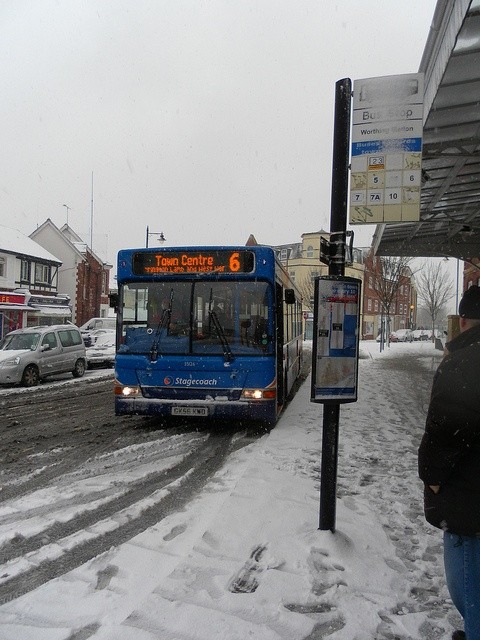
[{"left": 108, "top": 245, "right": 303, "bottom": 427}]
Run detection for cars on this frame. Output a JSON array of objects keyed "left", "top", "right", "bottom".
[
  {"left": 80, "top": 318, "right": 115, "bottom": 368},
  {"left": 0, "top": 324, "right": 88, "bottom": 388},
  {"left": 375, "top": 328, "right": 442, "bottom": 342}
]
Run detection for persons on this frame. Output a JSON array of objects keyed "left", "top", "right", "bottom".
[
  {"left": 418, "top": 287, "right": 479, "bottom": 639},
  {"left": 151, "top": 299, "right": 183, "bottom": 323}
]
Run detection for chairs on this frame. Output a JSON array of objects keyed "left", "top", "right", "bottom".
[{"left": 238, "top": 316, "right": 257, "bottom": 338}]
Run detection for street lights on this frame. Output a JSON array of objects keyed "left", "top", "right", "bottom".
[
  {"left": 146, "top": 225, "right": 166, "bottom": 248},
  {"left": 409, "top": 304, "right": 414, "bottom": 328}
]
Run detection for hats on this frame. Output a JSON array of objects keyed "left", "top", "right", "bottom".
[{"left": 459, "top": 284, "right": 480, "bottom": 320}]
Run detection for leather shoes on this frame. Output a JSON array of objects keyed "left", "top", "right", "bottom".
[{"left": 452, "top": 629, "right": 465, "bottom": 640}]
